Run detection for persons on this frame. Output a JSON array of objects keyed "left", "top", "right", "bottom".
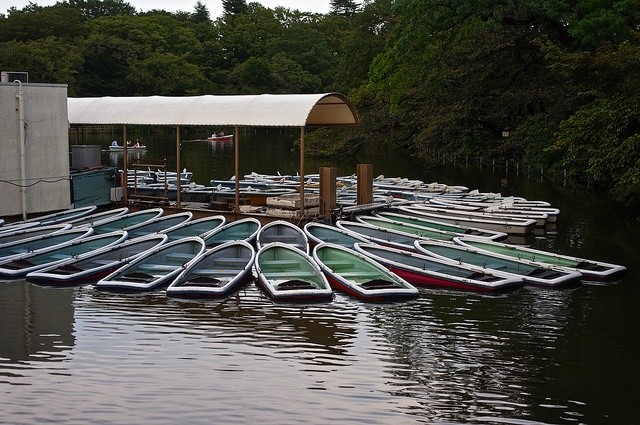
[
  {"left": 217, "top": 129, "right": 224, "bottom": 139},
  {"left": 211, "top": 130, "right": 218, "bottom": 140},
  {"left": 134, "top": 138, "right": 142, "bottom": 147},
  {"left": 112, "top": 138, "right": 118, "bottom": 148},
  {"left": 126, "top": 139, "right": 132, "bottom": 148}
]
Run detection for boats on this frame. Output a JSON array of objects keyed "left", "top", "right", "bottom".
[
  {"left": 204, "top": 218, "right": 261, "bottom": 251},
  {"left": 0, "top": 231, "right": 128, "bottom": 274},
  {"left": 371, "top": 211, "right": 508, "bottom": 239},
  {"left": 304, "top": 222, "right": 372, "bottom": 244},
  {"left": 0, "top": 221, "right": 40, "bottom": 233},
  {"left": 127, "top": 176, "right": 190, "bottom": 184},
  {"left": 402, "top": 183, "right": 468, "bottom": 197},
  {"left": 429, "top": 200, "right": 559, "bottom": 222},
  {"left": 354, "top": 242, "right": 524, "bottom": 291},
  {"left": 15, "top": 205, "right": 97, "bottom": 225},
  {"left": 313, "top": 243, "right": 419, "bottom": 300},
  {"left": 60, "top": 207, "right": 129, "bottom": 227},
  {"left": 412, "top": 204, "right": 548, "bottom": 225},
  {"left": 414, "top": 190, "right": 501, "bottom": 200},
  {"left": 373, "top": 178, "right": 428, "bottom": 189},
  {"left": 127, "top": 167, "right": 193, "bottom": 179},
  {"left": 110, "top": 146, "right": 146, "bottom": 150},
  {"left": 257, "top": 220, "right": 309, "bottom": 255},
  {"left": 0, "top": 228, "right": 94, "bottom": 260},
  {"left": 97, "top": 236, "right": 205, "bottom": 289},
  {"left": 80, "top": 208, "right": 163, "bottom": 232},
  {"left": 355, "top": 215, "right": 457, "bottom": 244},
  {"left": 252, "top": 242, "right": 332, "bottom": 299},
  {"left": 414, "top": 240, "right": 583, "bottom": 286},
  {"left": 123, "top": 212, "right": 193, "bottom": 234},
  {"left": 208, "top": 135, "right": 233, "bottom": 142},
  {"left": 400, "top": 206, "right": 537, "bottom": 233},
  {"left": 453, "top": 237, "right": 626, "bottom": 280},
  {"left": 437, "top": 196, "right": 551, "bottom": 207},
  {"left": 336, "top": 220, "right": 453, "bottom": 252},
  {"left": 167, "top": 240, "right": 256, "bottom": 294},
  {"left": 0, "top": 224, "right": 72, "bottom": 248},
  {"left": 161, "top": 215, "right": 225, "bottom": 238},
  {"left": 26, "top": 233, "right": 168, "bottom": 281},
  {"left": 244, "top": 172, "right": 292, "bottom": 181},
  {"left": 305, "top": 174, "right": 320, "bottom": 181},
  {"left": 336, "top": 174, "right": 358, "bottom": 184}
]
